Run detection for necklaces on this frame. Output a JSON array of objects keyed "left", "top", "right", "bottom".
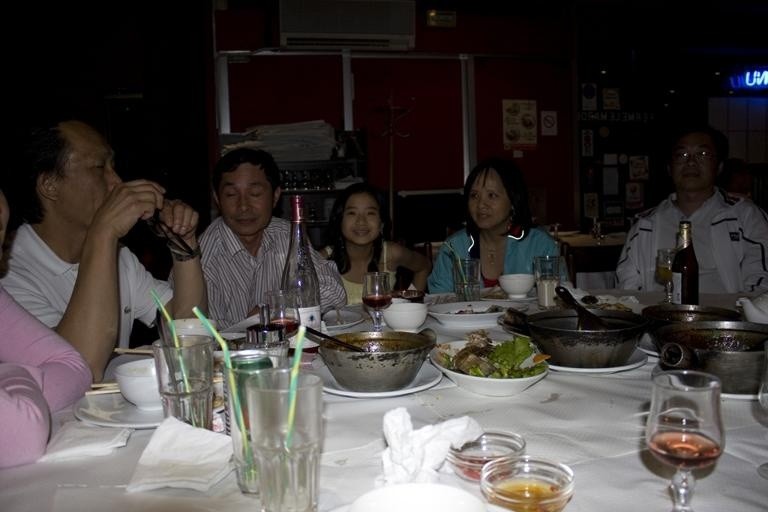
[{"left": 482, "top": 233, "right": 499, "bottom": 266}]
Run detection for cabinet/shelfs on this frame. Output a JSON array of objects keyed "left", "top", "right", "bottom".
[{"left": 222, "top": 130, "right": 368, "bottom": 259}]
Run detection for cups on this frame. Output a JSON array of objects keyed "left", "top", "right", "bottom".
[
  {"left": 151, "top": 333, "right": 214, "bottom": 434},
  {"left": 452, "top": 258, "right": 481, "bottom": 301},
  {"left": 536, "top": 255, "right": 561, "bottom": 310},
  {"left": 246, "top": 367, "right": 323, "bottom": 510},
  {"left": 221, "top": 353, "right": 292, "bottom": 498}
]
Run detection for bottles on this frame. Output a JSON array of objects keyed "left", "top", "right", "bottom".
[
  {"left": 670, "top": 220, "right": 699, "bottom": 307},
  {"left": 274, "top": 166, "right": 349, "bottom": 222},
  {"left": 278, "top": 192, "right": 323, "bottom": 357},
  {"left": 422, "top": 229, "right": 433, "bottom": 263}
]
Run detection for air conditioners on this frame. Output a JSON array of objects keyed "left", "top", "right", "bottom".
[{"left": 278, "top": 1, "right": 417, "bottom": 52}]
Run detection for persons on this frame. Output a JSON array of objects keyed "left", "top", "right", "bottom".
[
  {"left": 425, "top": 153, "right": 571, "bottom": 294},
  {"left": 0, "top": 160, "right": 93, "bottom": 470},
  {"left": 612, "top": 120, "right": 768, "bottom": 299},
  {"left": 167, "top": 145, "right": 348, "bottom": 330},
  {"left": 316, "top": 180, "right": 432, "bottom": 306},
  {"left": 717, "top": 156, "right": 753, "bottom": 199},
  {"left": 0, "top": 104, "right": 208, "bottom": 390}
]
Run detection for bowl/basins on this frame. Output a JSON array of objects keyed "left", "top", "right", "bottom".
[
  {"left": 377, "top": 303, "right": 426, "bottom": 335},
  {"left": 644, "top": 304, "right": 740, "bottom": 344},
  {"left": 447, "top": 428, "right": 526, "bottom": 483},
  {"left": 114, "top": 357, "right": 163, "bottom": 412},
  {"left": 653, "top": 319, "right": 766, "bottom": 395},
  {"left": 521, "top": 306, "right": 649, "bottom": 367},
  {"left": 479, "top": 453, "right": 577, "bottom": 512}
]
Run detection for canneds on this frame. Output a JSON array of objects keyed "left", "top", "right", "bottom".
[{"left": 230, "top": 353, "right": 275, "bottom": 436}]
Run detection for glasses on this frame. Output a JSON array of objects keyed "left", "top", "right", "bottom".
[{"left": 671, "top": 150, "right": 720, "bottom": 161}]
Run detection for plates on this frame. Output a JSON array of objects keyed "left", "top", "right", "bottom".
[
  {"left": 542, "top": 347, "right": 649, "bottom": 374},
  {"left": 432, "top": 341, "right": 550, "bottom": 398},
  {"left": 546, "top": 229, "right": 581, "bottom": 237},
  {"left": 425, "top": 299, "right": 525, "bottom": 328},
  {"left": 350, "top": 481, "right": 486, "bottom": 512},
  {"left": 309, "top": 353, "right": 442, "bottom": 400},
  {"left": 73, "top": 384, "right": 224, "bottom": 428},
  {"left": 317, "top": 304, "right": 359, "bottom": 333}
]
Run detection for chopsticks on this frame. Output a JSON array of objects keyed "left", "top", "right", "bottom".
[
  {"left": 85, "top": 375, "right": 225, "bottom": 396},
  {"left": 113, "top": 346, "right": 154, "bottom": 355}
]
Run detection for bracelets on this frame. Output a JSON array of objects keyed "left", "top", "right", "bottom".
[{"left": 170, "top": 239, "right": 202, "bottom": 262}]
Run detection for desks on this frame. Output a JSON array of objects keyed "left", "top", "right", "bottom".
[{"left": 417, "top": 231, "right": 626, "bottom": 288}]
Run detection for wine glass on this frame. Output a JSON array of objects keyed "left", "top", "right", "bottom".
[
  {"left": 264, "top": 288, "right": 300, "bottom": 349},
  {"left": 586, "top": 212, "right": 605, "bottom": 243},
  {"left": 644, "top": 368, "right": 726, "bottom": 512},
  {"left": 755, "top": 369, "right": 768, "bottom": 480},
  {"left": 363, "top": 271, "right": 392, "bottom": 332},
  {"left": 655, "top": 248, "right": 677, "bottom": 305},
  {"left": 548, "top": 223, "right": 562, "bottom": 247}
]
